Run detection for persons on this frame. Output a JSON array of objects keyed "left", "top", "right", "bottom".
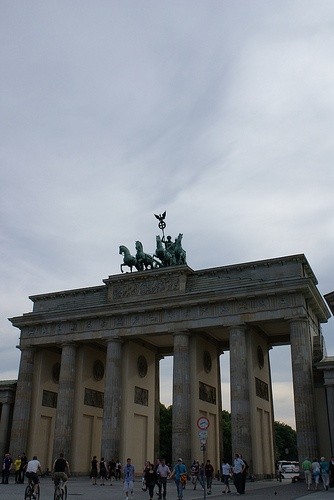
[
  {"left": 319, "top": 457, "right": 330, "bottom": 491},
  {"left": 161, "top": 236, "right": 173, "bottom": 249},
  {"left": 240, "top": 454, "right": 249, "bottom": 494},
  {"left": 89, "top": 456, "right": 122, "bottom": 486},
  {"left": 232, "top": 453, "right": 246, "bottom": 495},
  {"left": 189, "top": 460, "right": 214, "bottom": 495},
  {"left": 0, "top": 452, "right": 27, "bottom": 484},
  {"left": 276, "top": 465, "right": 286, "bottom": 482},
  {"left": 312, "top": 459, "right": 321, "bottom": 491},
  {"left": 51, "top": 453, "right": 70, "bottom": 497},
  {"left": 23, "top": 456, "right": 42, "bottom": 499},
  {"left": 330, "top": 461, "right": 334, "bottom": 489},
  {"left": 123, "top": 458, "right": 135, "bottom": 500},
  {"left": 141, "top": 458, "right": 190, "bottom": 500},
  {"left": 220, "top": 458, "right": 232, "bottom": 494},
  {"left": 301, "top": 457, "right": 311, "bottom": 490}
]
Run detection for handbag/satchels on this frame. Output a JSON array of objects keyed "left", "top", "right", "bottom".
[
  {"left": 142, "top": 484, "right": 147, "bottom": 491},
  {"left": 180, "top": 477, "right": 184, "bottom": 483}
]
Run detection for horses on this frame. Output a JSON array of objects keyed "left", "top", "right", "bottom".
[
  {"left": 119, "top": 245, "right": 135, "bottom": 273},
  {"left": 134, "top": 241, "right": 154, "bottom": 270},
  {"left": 153, "top": 233, "right": 186, "bottom": 265}
]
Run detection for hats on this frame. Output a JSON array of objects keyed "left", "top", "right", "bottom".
[{"left": 178, "top": 458, "right": 183, "bottom": 462}]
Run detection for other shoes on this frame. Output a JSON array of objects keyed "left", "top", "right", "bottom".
[
  {"left": 92, "top": 478, "right": 120, "bottom": 486},
  {"left": 222, "top": 488, "right": 245, "bottom": 495},
  {"left": 1, "top": 480, "right": 24, "bottom": 485},
  {"left": 193, "top": 485, "right": 214, "bottom": 495},
  {"left": 307, "top": 484, "right": 329, "bottom": 491}
]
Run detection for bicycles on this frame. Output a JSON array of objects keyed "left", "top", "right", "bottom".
[
  {"left": 54, "top": 476, "right": 71, "bottom": 500},
  {"left": 25, "top": 474, "right": 40, "bottom": 500}
]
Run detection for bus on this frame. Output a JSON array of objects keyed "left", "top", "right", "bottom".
[{"left": 277, "top": 461, "right": 299, "bottom": 473}]
